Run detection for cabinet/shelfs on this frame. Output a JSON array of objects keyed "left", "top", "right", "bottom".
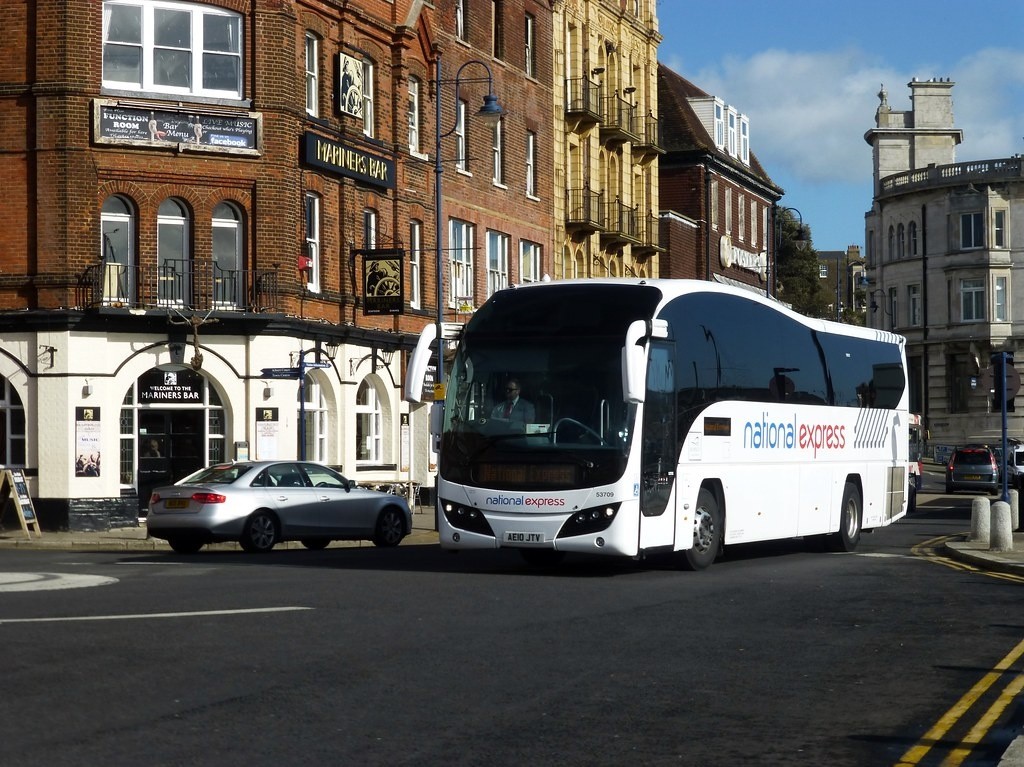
[{"left": 104, "top": 263, "right": 121, "bottom": 300}]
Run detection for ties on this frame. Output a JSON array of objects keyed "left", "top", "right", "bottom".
[{"left": 504, "top": 402, "right": 512, "bottom": 416}]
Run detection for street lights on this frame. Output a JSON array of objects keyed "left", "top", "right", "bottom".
[
  {"left": 835, "top": 255, "right": 873, "bottom": 323},
  {"left": 432, "top": 55, "right": 508, "bottom": 531},
  {"left": 766, "top": 204, "right": 806, "bottom": 298}
]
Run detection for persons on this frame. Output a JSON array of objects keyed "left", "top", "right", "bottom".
[
  {"left": 145, "top": 439, "right": 161, "bottom": 457},
  {"left": 489, "top": 378, "right": 536, "bottom": 425},
  {"left": 78, "top": 451, "right": 100, "bottom": 476}
]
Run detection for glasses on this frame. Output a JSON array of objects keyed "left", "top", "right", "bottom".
[{"left": 507, "top": 388, "right": 517, "bottom": 391}]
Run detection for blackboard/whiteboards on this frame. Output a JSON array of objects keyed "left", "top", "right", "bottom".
[{"left": 6, "top": 469, "right": 37, "bottom": 523}]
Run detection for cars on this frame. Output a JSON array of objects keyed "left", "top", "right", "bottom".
[
  {"left": 1002, "top": 444, "right": 1024, "bottom": 488},
  {"left": 145, "top": 458, "right": 414, "bottom": 557},
  {"left": 941, "top": 444, "right": 999, "bottom": 495}
]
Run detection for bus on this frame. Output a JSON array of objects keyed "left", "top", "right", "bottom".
[
  {"left": 401, "top": 274, "right": 912, "bottom": 574},
  {"left": 908, "top": 412, "right": 930, "bottom": 513}
]
